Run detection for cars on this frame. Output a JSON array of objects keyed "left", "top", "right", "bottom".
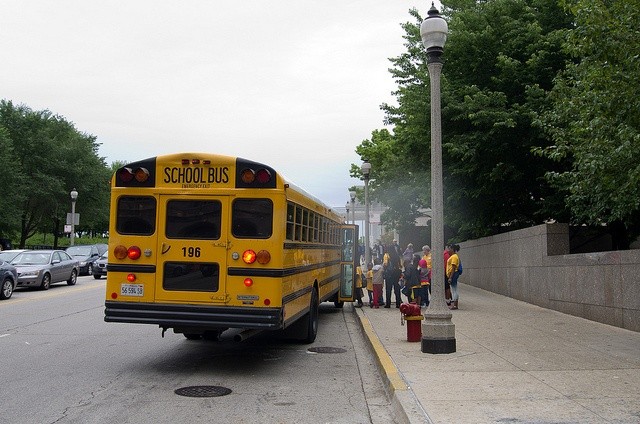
[
  {"left": 95, "top": 243, "right": 107, "bottom": 254},
  {"left": 66, "top": 245, "right": 100, "bottom": 276},
  {"left": 92, "top": 249, "right": 111, "bottom": 280},
  {"left": 8, "top": 250, "right": 80, "bottom": 290},
  {"left": 0, "top": 249, "right": 31, "bottom": 276},
  {"left": 0, "top": 258, "right": 18, "bottom": 300}
]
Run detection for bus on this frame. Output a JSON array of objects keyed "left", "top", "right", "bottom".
[{"left": 103, "top": 152, "right": 359, "bottom": 347}]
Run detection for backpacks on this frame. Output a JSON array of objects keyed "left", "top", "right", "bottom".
[{"left": 388, "top": 264, "right": 402, "bottom": 284}]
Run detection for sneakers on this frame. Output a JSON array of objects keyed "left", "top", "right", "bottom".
[{"left": 450, "top": 305, "right": 458, "bottom": 309}]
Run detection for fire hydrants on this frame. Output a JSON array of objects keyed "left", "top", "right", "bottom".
[{"left": 399, "top": 298, "right": 427, "bottom": 343}]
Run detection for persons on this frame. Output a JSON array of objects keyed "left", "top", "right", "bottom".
[
  {"left": 383, "top": 247, "right": 403, "bottom": 308},
  {"left": 402, "top": 243, "right": 414, "bottom": 259},
  {"left": 446, "top": 243, "right": 462, "bottom": 310},
  {"left": 417, "top": 245, "right": 431, "bottom": 307},
  {"left": 444, "top": 244, "right": 453, "bottom": 306},
  {"left": 378, "top": 242, "right": 384, "bottom": 260},
  {"left": 371, "top": 259, "right": 384, "bottom": 308},
  {"left": 418, "top": 259, "right": 429, "bottom": 309},
  {"left": 393, "top": 241, "right": 401, "bottom": 266},
  {"left": 401, "top": 260, "right": 421, "bottom": 304},
  {"left": 365, "top": 262, "right": 373, "bottom": 306},
  {"left": 355, "top": 265, "right": 364, "bottom": 308}
]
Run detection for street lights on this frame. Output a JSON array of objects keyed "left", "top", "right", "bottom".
[
  {"left": 360, "top": 157, "right": 373, "bottom": 307},
  {"left": 418, "top": 1, "right": 458, "bottom": 355},
  {"left": 69, "top": 186, "right": 79, "bottom": 248},
  {"left": 349, "top": 187, "right": 357, "bottom": 226},
  {"left": 345, "top": 200, "right": 351, "bottom": 226}
]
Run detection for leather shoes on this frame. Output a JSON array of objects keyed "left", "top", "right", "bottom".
[
  {"left": 383, "top": 305, "right": 390, "bottom": 308},
  {"left": 396, "top": 304, "right": 400, "bottom": 307}
]
